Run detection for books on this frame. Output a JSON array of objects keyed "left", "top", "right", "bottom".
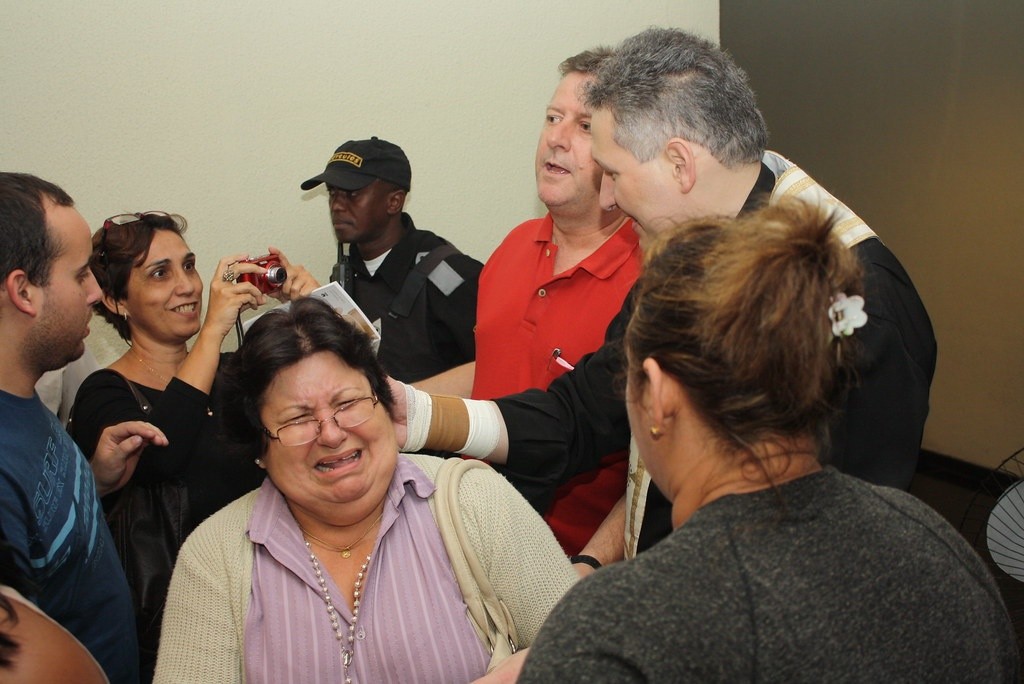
[{"left": 243, "top": 282, "right": 380, "bottom": 345}]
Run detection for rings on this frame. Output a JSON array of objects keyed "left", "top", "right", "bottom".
[{"left": 223, "top": 271, "right": 234, "bottom": 282}]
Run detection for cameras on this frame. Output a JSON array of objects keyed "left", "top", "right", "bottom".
[{"left": 237, "top": 254, "right": 288, "bottom": 295}]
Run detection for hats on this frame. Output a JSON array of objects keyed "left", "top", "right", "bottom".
[{"left": 301, "top": 136, "right": 411, "bottom": 192}]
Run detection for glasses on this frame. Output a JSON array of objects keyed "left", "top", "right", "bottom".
[
  {"left": 99, "top": 210, "right": 169, "bottom": 268},
  {"left": 253, "top": 385, "right": 378, "bottom": 447}
]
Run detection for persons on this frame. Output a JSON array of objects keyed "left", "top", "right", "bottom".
[
  {"left": 514, "top": 215, "right": 1023, "bottom": 683},
  {"left": 68, "top": 210, "right": 324, "bottom": 683},
  {"left": 380, "top": 29, "right": 936, "bottom": 554},
  {"left": 408, "top": 48, "right": 645, "bottom": 574},
  {"left": 301, "top": 137, "right": 485, "bottom": 400},
  {"left": 151, "top": 298, "right": 585, "bottom": 684},
  {"left": 1, "top": 172, "right": 171, "bottom": 683}
]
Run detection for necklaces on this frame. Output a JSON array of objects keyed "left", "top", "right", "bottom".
[
  {"left": 297, "top": 514, "right": 383, "bottom": 557},
  {"left": 304, "top": 540, "right": 371, "bottom": 684},
  {"left": 130, "top": 347, "right": 169, "bottom": 384}
]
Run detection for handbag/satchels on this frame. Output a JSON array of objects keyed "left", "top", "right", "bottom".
[{"left": 64, "top": 368, "right": 189, "bottom": 654}]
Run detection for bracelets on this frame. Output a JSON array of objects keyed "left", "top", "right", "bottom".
[{"left": 571, "top": 556, "right": 600, "bottom": 569}]
